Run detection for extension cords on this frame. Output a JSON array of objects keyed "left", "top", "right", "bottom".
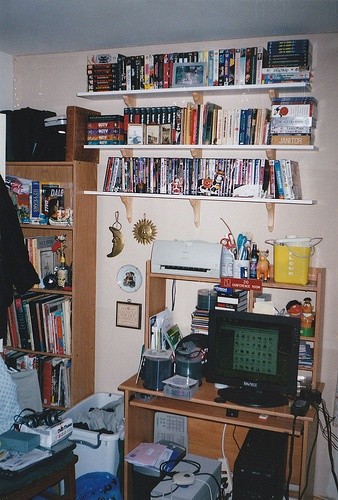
[{"left": 217, "top": 457, "right": 232, "bottom": 493}]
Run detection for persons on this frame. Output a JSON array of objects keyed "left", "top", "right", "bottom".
[{"left": 299, "top": 298, "right": 316, "bottom": 328}]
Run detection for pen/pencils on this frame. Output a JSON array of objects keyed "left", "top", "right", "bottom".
[{"left": 239, "top": 240, "right": 251, "bottom": 260}]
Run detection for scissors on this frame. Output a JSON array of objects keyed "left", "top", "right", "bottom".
[
  {"left": 220, "top": 233, "right": 236, "bottom": 252},
  {"left": 237, "top": 233, "right": 246, "bottom": 251}
]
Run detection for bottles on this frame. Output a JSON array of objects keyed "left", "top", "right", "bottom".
[
  {"left": 143, "top": 356, "right": 172, "bottom": 391},
  {"left": 247, "top": 242, "right": 270, "bottom": 281},
  {"left": 57, "top": 254, "right": 69, "bottom": 288},
  {"left": 175, "top": 356, "right": 202, "bottom": 386}
]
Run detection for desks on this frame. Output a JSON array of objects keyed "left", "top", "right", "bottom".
[{"left": 0, "top": 454, "right": 79, "bottom": 500}]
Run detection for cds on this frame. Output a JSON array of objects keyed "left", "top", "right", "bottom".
[
  {"left": 196, "top": 290, "right": 217, "bottom": 309},
  {"left": 142, "top": 355, "right": 202, "bottom": 391}
]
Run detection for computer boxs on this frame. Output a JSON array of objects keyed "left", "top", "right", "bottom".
[
  {"left": 233, "top": 426, "right": 289, "bottom": 500},
  {"left": 150, "top": 453, "right": 222, "bottom": 500}
]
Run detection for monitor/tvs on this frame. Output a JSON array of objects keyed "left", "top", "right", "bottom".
[{"left": 205, "top": 309, "right": 300, "bottom": 407}]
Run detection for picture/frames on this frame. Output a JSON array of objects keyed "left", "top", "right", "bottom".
[{"left": 116, "top": 301, "right": 142, "bottom": 329}]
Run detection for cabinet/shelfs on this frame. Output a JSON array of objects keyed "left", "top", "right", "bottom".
[
  {"left": 117, "top": 259, "right": 327, "bottom": 500},
  {"left": 75, "top": 82, "right": 317, "bottom": 205},
  {"left": 6, "top": 160, "right": 97, "bottom": 410}
]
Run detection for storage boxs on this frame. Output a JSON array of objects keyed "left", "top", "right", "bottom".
[{"left": 55, "top": 392, "right": 123, "bottom": 500}]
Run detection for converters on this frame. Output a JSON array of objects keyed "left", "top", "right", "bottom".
[{"left": 290, "top": 398, "right": 310, "bottom": 417}]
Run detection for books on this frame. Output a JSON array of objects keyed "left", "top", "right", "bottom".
[
  {"left": 0, "top": 173, "right": 72, "bottom": 411},
  {"left": 190, "top": 285, "right": 247, "bottom": 335},
  {"left": 299, "top": 340, "right": 314, "bottom": 370},
  {"left": 80, "top": 38, "right": 321, "bottom": 201}
]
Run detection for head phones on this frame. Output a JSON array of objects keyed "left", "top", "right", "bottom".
[{"left": 26, "top": 410, "right": 58, "bottom": 428}]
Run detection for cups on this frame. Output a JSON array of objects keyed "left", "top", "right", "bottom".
[{"left": 233, "top": 260, "right": 250, "bottom": 278}]
